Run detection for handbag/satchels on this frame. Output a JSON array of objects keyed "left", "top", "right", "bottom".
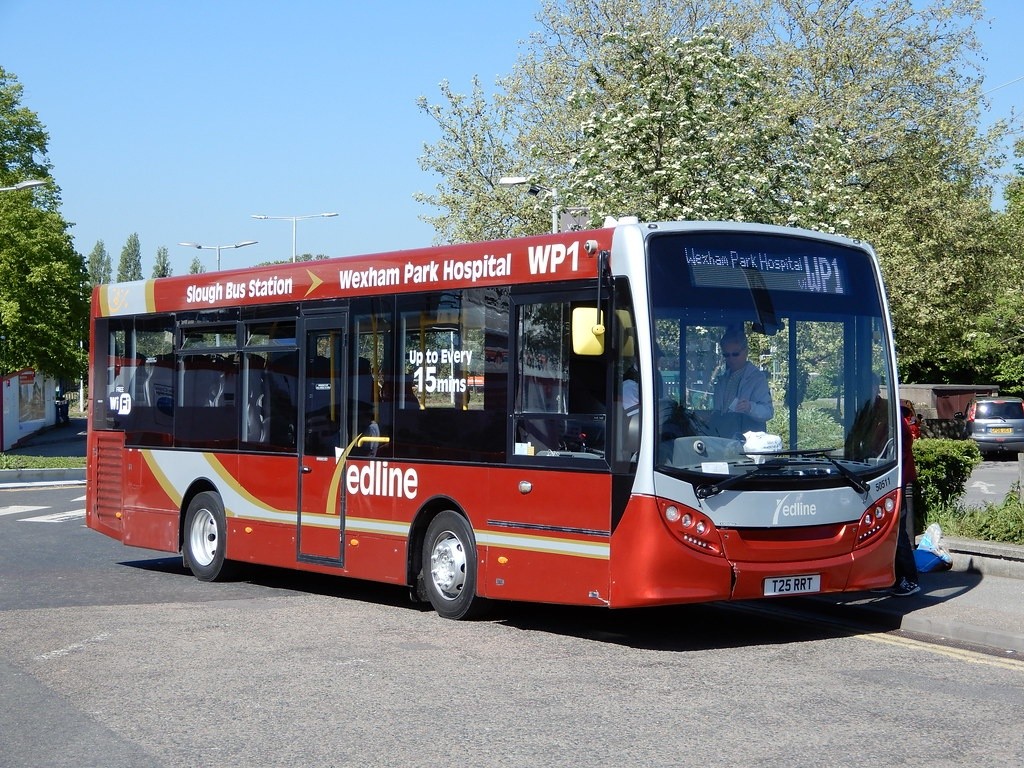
[{"left": 913, "top": 522, "right": 953, "bottom": 573}]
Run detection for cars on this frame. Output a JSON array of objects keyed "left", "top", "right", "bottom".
[
  {"left": 955, "top": 396, "right": 1024, "bottom": 454},
  {"left": 899, "top": 399, "right": 924, "bottom": 440}
]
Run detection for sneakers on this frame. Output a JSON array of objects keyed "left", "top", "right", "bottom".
[{"left": 894, "top": 581, "right": 921, "bottom": 595}]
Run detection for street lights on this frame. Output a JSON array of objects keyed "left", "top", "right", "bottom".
[
  {"left": 176, "top": 241, "right": 258, "bottom": 271},
  {"left": 498, "top": 177, "right": 559, "bottom": 233},
  {"left": 251, "top": 212, "right": 339, "bottom": 262}
]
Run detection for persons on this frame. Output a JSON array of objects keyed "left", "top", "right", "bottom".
[
  {"left": 263, "top": 325, "right": 320, "bottom": 451},
  {"left": 623, "top": 344, "right": 679, "bottom": 417},
  {"left": 872, "top": 372, "right": 921, "bottom": 595},
  {"left": 714, "top": 332, "right": 774, "bottom": 433},
  {"left": 380, "top": 375, "right": 419, "bottom": 437}
]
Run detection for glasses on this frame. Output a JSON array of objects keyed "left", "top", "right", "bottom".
[{"left": 722, "top": 348, "right": 742, "bottom": 358}]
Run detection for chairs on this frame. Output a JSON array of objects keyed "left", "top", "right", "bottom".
[{"left": 113, "top": 348, "right": 419, "bottom": 437}]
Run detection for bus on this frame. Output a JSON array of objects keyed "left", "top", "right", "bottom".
[{"left": 86, "top": 219, "right": 905, "bottom": 619}]
